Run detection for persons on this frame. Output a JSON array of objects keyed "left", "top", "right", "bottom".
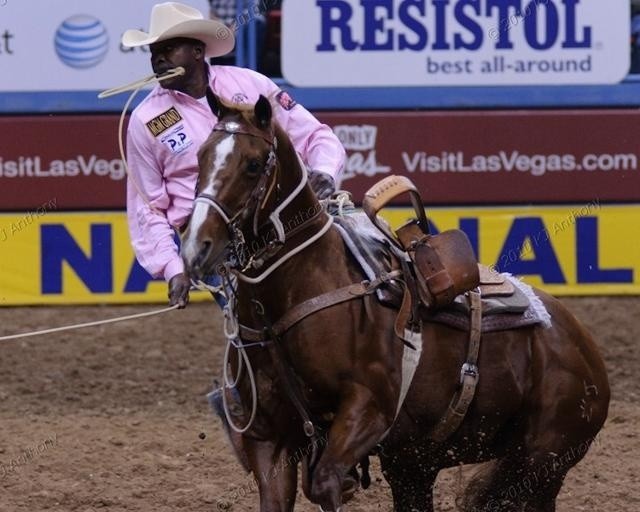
[
  {"left": 208, "top": 0, "right": 282, "bottom": 79},
  {"left": 121, "top": 0, "right": 412, "bottom": 506}
]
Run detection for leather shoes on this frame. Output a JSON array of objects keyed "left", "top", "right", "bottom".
[{"left": 207, "top": 382, "right": 253, "bottom": 476}]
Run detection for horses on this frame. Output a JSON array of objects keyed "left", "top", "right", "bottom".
[{"left": 176, "top": 84, "right": 612, "bottom": 512}]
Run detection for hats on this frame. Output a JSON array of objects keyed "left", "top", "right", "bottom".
[{"left": 121, "top": 3, "right": 235, "bottom": 59}]
